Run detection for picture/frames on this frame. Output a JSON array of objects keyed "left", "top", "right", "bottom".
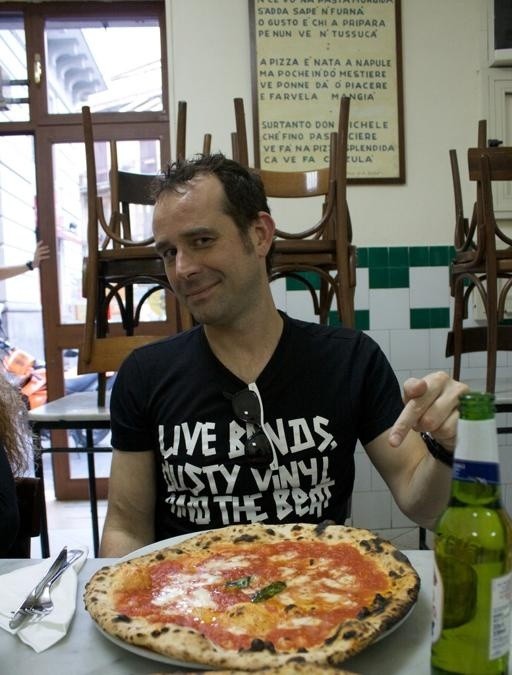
[{"left": 246, "top": 0, "right": 408, "bottom": 185}]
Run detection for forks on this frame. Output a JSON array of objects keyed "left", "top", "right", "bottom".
[{"left": 17, "top": 549, "right": 85, "bottom": 615}]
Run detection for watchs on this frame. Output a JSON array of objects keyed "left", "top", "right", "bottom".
[{"left": 95, "top": 150, "right": 472, "bottom": 559}]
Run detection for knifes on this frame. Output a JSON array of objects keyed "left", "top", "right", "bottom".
[{"left": 7, "top": 545, "right": 68, "bottom": 632}]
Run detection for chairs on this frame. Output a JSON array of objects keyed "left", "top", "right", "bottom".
[{"left": 444, "top": 117, "right": 511, "bottom": 402}]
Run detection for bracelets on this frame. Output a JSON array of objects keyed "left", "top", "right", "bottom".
[
  {"left": 25, "top": 261, "right": 33, "bottom": 270},
  {"left": 420, "top": 432, "right": 454, "bottom": 469}
]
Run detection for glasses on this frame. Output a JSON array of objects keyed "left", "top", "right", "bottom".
[{"left": 230, "top": 382, "right": 279, "bottom": 472}]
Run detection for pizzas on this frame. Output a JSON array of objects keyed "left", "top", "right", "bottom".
[{"left": 158, "top": 661, "right": 359, "bottom": 675}]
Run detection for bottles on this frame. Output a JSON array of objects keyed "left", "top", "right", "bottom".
[{"left": 432, "top": 390, "right": 512, "bottom": 675}]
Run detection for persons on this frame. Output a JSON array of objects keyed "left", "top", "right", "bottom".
[
  {"left": 0, "top": 364, "right": 42, "bottom": 558},
  {"left": 0, "top": 240, "right": 51, "bottom": 280}
]
